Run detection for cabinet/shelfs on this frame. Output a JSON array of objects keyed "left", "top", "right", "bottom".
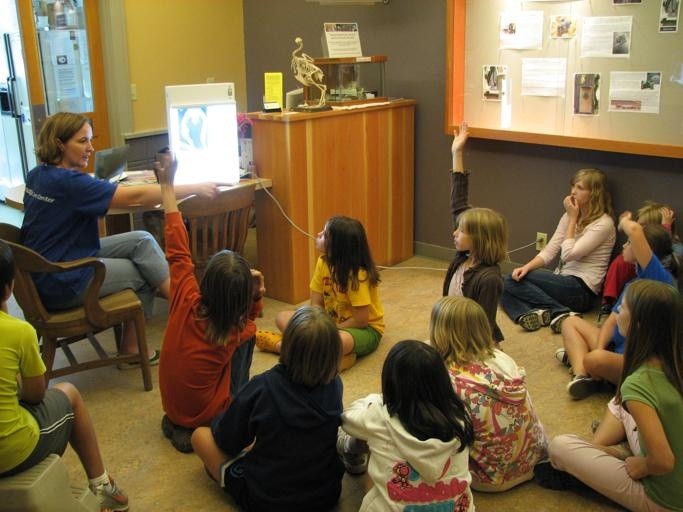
[{"left": 246, "top": 97, "right": 417, "bottom": 303}]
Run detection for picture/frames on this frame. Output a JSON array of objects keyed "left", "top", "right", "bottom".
[{"left": 445, "top": 0, "right": 683, "bottom": 159}]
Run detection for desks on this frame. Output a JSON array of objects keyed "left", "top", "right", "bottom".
[{"left": 6, "top": 171, "right": 273, "bottom": 352}]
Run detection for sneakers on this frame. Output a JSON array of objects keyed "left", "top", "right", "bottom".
[
  {"left": 556, "top": 348, "right": 572, "bottom": 367},
  {"left": 337, "top": 426, "right": 368, "bottom": 474},
  {"left": 534, "top": 461, "right": 577, "bottom": 492},
  {"left": 161, "top": 415, "right": 195, "bottom": 452},
  {"left": 519, "top": 309, "right": 550, "bottom": 330},
  {"left": 116, "top": 350, "right": 160, "bottom": 370},
  {"left": 550, "top": 312, "right": 583, "bottom": 333},
  {"left": 567, "top": 377, "right": 604, "bottom": 398},
  {"left": 89, "top": 475, "right": 128, "bottom": 512}
]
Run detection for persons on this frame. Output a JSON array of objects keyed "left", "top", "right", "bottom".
[
  {"left": 0, "top": 240, "right": 129, "bottom": 511},
  {"left": 442, "top": 122, "right": 506, "bottom": 350},
  {"left": 254, "top": 215, "right": 387, "bottom": 371},
  {"left": 426, "top": 295, "right": 550, "bottom": 493},
  {"left": 189, "top": 304, "right": 346, "bottom": 511},
  {"left": 498, "top": 165, "right": 681, "bottom": 512},
  {"left": 17, "top": 111, "right": 235, "bottom": 371},
  {"left": 152, "top": 149, "right": 266, "bottom": 437},
  {"left": 336, "top": 338, "right": 479, "bottom": 511}
]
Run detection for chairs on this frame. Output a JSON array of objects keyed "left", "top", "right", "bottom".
[
  {"left": 0, "top": 220, "right": 152, "bottom": 392},
  {"left": 143, "top": 182, "right": 256, "bottom": 281}
]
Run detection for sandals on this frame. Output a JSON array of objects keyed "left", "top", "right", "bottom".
[
  {"left": 338, "top": 354, "right": 356, "bottom": 371},
  {"left": 255, "top": 329, "right": 282, "bottom": 353}
]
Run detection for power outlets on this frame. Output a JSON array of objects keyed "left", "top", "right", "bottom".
[{"left": 536, "top": 231, "right": 547, "bottom": 251}]
